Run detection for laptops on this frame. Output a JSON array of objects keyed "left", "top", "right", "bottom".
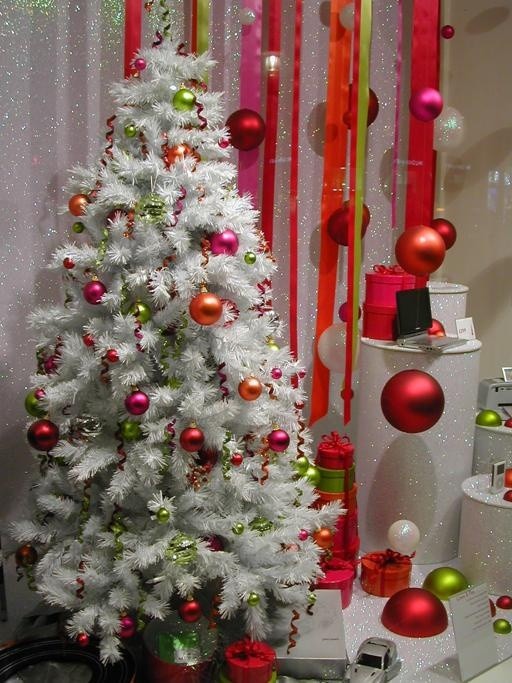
[{"left": 396, "top": 288, "right": 468, "bottom": 354}]
